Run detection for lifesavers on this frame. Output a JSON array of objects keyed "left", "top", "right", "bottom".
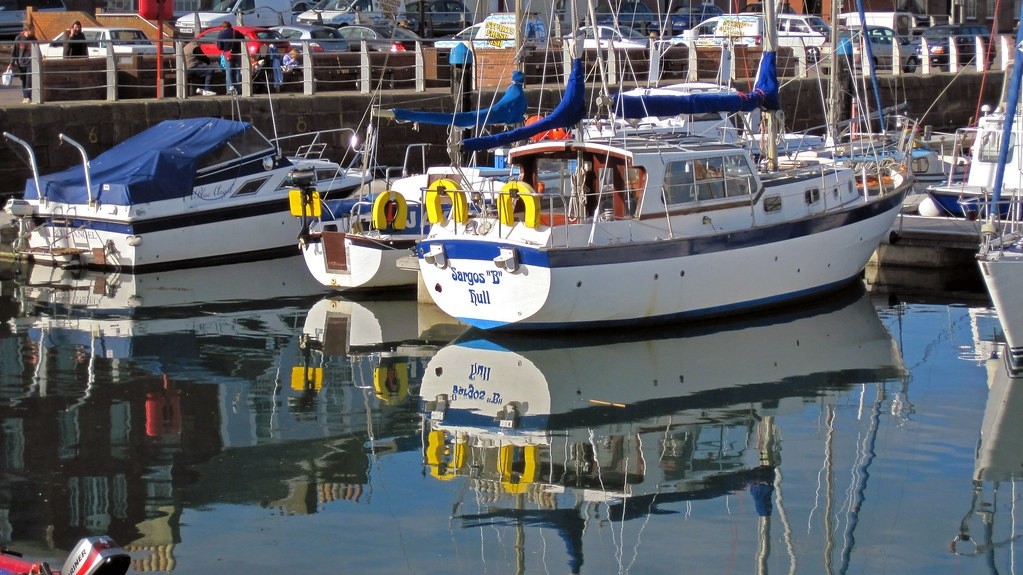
[
  {"left": 497, "top": 181, "right": 540, "bottom": 228},
  {"left": 496, "top": 447, "right": 540, "bottom": 494},
  {"left": 372, "top": 191, "right": 408, "bottom": 230},
  {"left": 424, "top": 179, "right": 467, "bottom": 224},
  {"left": 372, "top": 362, "right": 410, "bottom": 404},
  {"left": 427, "top": 431, "right": 468, "bottom": 482}
]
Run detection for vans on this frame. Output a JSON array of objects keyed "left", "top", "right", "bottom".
[
  {"left": 296, "top": 0, "right": 407, "bottom": 29},
  {"left": 448, "top": 17, "right": 549, "bottom": 48},
  {"left": 910, "top": 24, "right": 997, "bottom": 73}
]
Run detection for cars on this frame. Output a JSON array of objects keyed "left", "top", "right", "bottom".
[
  {"left": 337, "top": 25, "right": 434, "bottom": 51},
  {"left": 405, "top": 0, "right": 472, "bottom": 38},
  {"left": 190, "top": 26, "right": 292, "bottom": 65},
  {"left": 560, "top": 25, "right": 650, "bottom": 49},
  {"left": 268, "top": 25, "right": 352, "bottom": 53},
  {"left": 738, "top": 3, "right": 796, "bottom": 14},
  {"left": 578, "top": 1, "right": 653, "bottom": 35},
  {"left": 819, "top": 25, "right": 919, "bottom": 75},
  {"left": 648, "top": 5, "right": 726, "bottom": 36},
  {"left": 38, "top": 27, "right": 176, "bottom": 64}
]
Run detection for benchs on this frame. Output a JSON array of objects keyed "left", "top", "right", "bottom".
[
  {"left": 163, "top": 69, "right": 226, "bottom": 96},
  {"left": 660, "top": 56, "right": 689, "bottom": 77},
  {"left": 334, "top": 65, "right": 396, "bottom": 87}
]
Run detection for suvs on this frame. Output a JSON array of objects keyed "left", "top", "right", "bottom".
[
  {"left": 0, "top": 0, "right": 66, "bottom": 35},
  {"left": 775, "top": 13, "right": 829, "bottom": 63}
]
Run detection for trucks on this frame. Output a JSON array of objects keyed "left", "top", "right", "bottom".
[
  {"left": 175, "top": 0, "right": 292, "bottom": 39},
  {"left": 838, "top": 12, "right": 912, "bottom": 37},
  {"left": 677, "top": 16, "right": 764, "bottom": 46}
]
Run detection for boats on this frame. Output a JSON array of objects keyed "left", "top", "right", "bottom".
[
  {"left": 284, "top": 0, "right": 1023, "bottom": 360},
  {"left": 2, "top": 71, "right": 373, "bottom": 268}
]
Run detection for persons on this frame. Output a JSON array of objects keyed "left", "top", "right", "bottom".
[
  {"left": 217, "top": 21, "right": 246, "bottom": 95},
  {"left": 183, "top": 42, "right": 217, "bottom": 96},
  {"left": 63, "top": 21, "right": 88, "bottom": 59},
  {"left": 8, "top": 23, "right": 39, "bottom": 103},
  {"left": 283, "top": 48, "right": 303, "bottom": 77}
]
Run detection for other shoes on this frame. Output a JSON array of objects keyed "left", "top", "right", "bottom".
[
  {"left": 202, "top": 90, "right": 216, "bottom": 96},
  {"left": 22, "top": 97, "right": 31, "bottom": 103},
  {"left": 196, "top": 88, "right": 204, "bottom": 94}
]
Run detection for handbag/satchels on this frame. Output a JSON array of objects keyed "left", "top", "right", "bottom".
[
  {"left": 225, "top": 51, "right": 233, "bottom": 60},
  {"left": 2, "top": 68, "right": 12, "bottom": 86}
]
